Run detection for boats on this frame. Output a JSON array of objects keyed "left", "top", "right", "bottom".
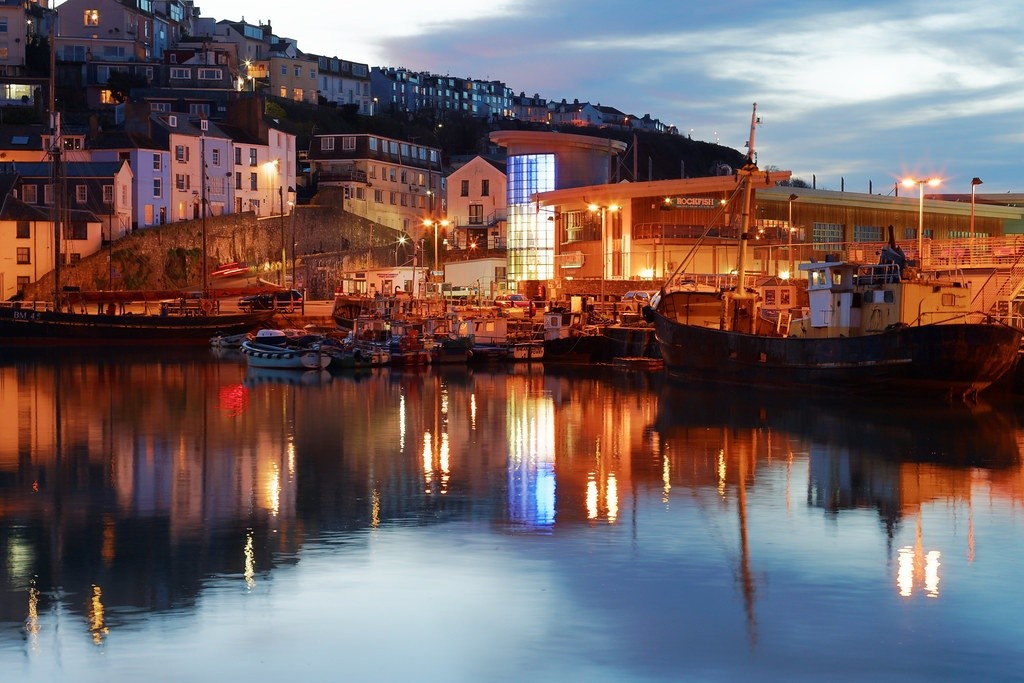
[
  {"left": 642, "top": 101, "right": 1024, "bottom": 419},
  {"left": 205, "top": 238, "right": 658, "bottom": 371}
]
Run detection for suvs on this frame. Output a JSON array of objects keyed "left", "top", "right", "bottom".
[{"left": 237, "top": 289, "right": 304, "bottom": 314}]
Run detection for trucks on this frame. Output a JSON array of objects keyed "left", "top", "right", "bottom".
[{"left": 426, "top": 282, "right": 479, "bottom": 306}]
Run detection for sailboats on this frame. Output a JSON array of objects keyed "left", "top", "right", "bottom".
[{"left": 0, "top": 1, "right": 288, "bottom": 350}]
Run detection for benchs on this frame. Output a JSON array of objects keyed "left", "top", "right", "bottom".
[{"left": 168, "top": 307, "right": 200, "bottom": 317}]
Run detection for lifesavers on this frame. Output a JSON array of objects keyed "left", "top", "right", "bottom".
[
  {"left": 202, "top": 300, "right": 212, "bottom": 312},
  {"left": 570, "top": 323, "right": 583, "bottom": 337}
]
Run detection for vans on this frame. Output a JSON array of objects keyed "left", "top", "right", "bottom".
[{"left": 620, "top": 289, "right": 660, "bottom": 313}]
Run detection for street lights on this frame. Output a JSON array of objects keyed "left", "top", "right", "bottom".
[
  {"left": 902, "top": 176, "right": 943, "bottom": 266},
  {"left": 787, "top": 192, "right": 799, "bottom": 280},
  {"left": 587, "top": 204, "right": 621, "bottom": 316},
  {"left": 659, "top": 205, "right": 673, "bottom": 278},
  {"left": 423, "top": 219, "right": 450, "bottom": 312},
  {"left": 394, "top": 235, "right": 406, "bottom": 267},
  {"left": 426, "top": 191, "right": 435, "bottom": 220},
  {"left": 968, "top": 176, "right": 983, "bottom": 266}
]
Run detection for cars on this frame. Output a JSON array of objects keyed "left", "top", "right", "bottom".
[
  {"left": 158, "top": 291, "right": 218, "bottom": 315},
  {"left": 493, "top": 293, "right": 537, "bottom": 317}
]
